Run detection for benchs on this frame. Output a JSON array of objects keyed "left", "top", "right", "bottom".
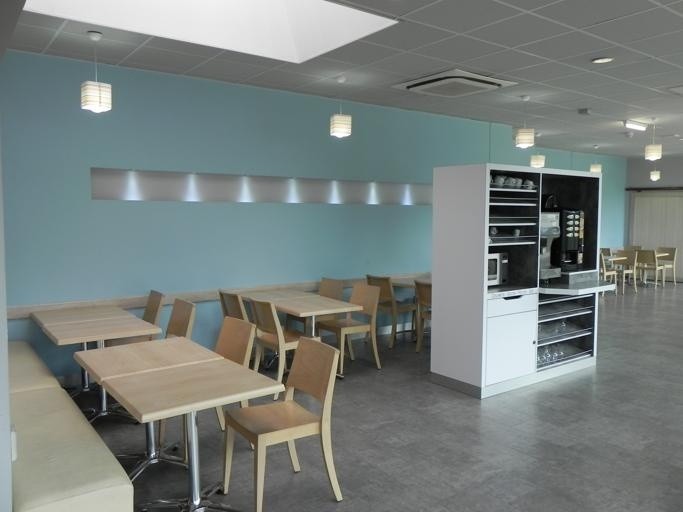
[{"left": 6, "top": 337, "right": 135, "bottom": 510}]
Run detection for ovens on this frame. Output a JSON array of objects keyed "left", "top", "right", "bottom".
[{"left": 488, "top": 253, "right": 509, "bottom": 287}]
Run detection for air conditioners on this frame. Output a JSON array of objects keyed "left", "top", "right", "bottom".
[{"left": 390, "top": 68, "right": 520, "bottom": 99}]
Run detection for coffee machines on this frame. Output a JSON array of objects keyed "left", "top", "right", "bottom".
[
  {"left": 540, "top": 212, "right": 561, "bottom": 284},
  {"left": 559, "top": 209, "right": 585, "bottom": 271}
]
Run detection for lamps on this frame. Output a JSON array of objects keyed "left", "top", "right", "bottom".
[
  {"left": 643, "top": 117, "right": 662, "bottom": 162},
  {"left": 514, "top": 94, "right": 535, "bottom": 151},
  {"left": 80, "top": 30, "right": 113, "bottom": 115},
  {"left": 329, "top": 73, "right": 352, "bottom": 139},
  {"left": 529, "top": 131, "right": 545, "bottom": 169},
  {"left": 651, "top": 164, "right": 662, "bottom": 182},
  {"left": 589, "top": 142, "right": 603, "bottom": 173}
]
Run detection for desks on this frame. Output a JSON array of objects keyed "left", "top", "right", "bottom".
[
  {"left": 72, "top": 337, "right": 225, "bottom": 482},
  {"left": 30, "top": 305, "right": 138, "bottom": 399},
  {"left": 41, "top": 316, "right": 163, "bottom": 426},
  {"left": 274, "top": 295, "right": 364, "bottom": 339},
  {"left": 100, "top": 357, "right": 287, "bottom": 511}
]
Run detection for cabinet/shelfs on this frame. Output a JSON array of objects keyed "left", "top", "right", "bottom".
[{"left": 427, "top": 162, "right": 602, "bottom": 401}]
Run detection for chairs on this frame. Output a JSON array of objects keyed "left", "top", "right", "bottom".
[
  {"left": 413, "top": 279, "right": 432, "bottom": 353},
  {"left": 165, "top": 297, "right": 196, "bottom": 339},
  {"left": 600, "top": 247, "right": 614, "bottom": 271},
  {"left": 600, "top": 257, "right": 618, "bottom": 297},
  {"left": 634, "top": 250, "right": 665, "bottom": 289},
  {"left": 222, "top": 335, "right": 344, "bottom": 511},
  {"left": 655, "top": 246, "right": 678, "bottom": 286},
  {"left": 366, "top": 272, "right": 417, "bottom": 349},
  {"left": 158, "top": 315, "right": 257, "bottom": 451},
  {"left": 618, "top": 249, "right": 638, "bottom": 295},
  {"left": 217, "top": 287, "right": 265, "bottom": 362},
  {"left": 108, "top": 289, "right": 165, "bottom": 346},
  {"left": 240, "top": 295, "right": 321, "bottom": 402},
  {"left": 285, "top": 277, "right": 344, "bottom": 336},
  {"left": 315, "top": 283, "right": 382, "bottom": 381},
  {"left": 624, "top": 245, "right": 641, "bottom": 251}
]
